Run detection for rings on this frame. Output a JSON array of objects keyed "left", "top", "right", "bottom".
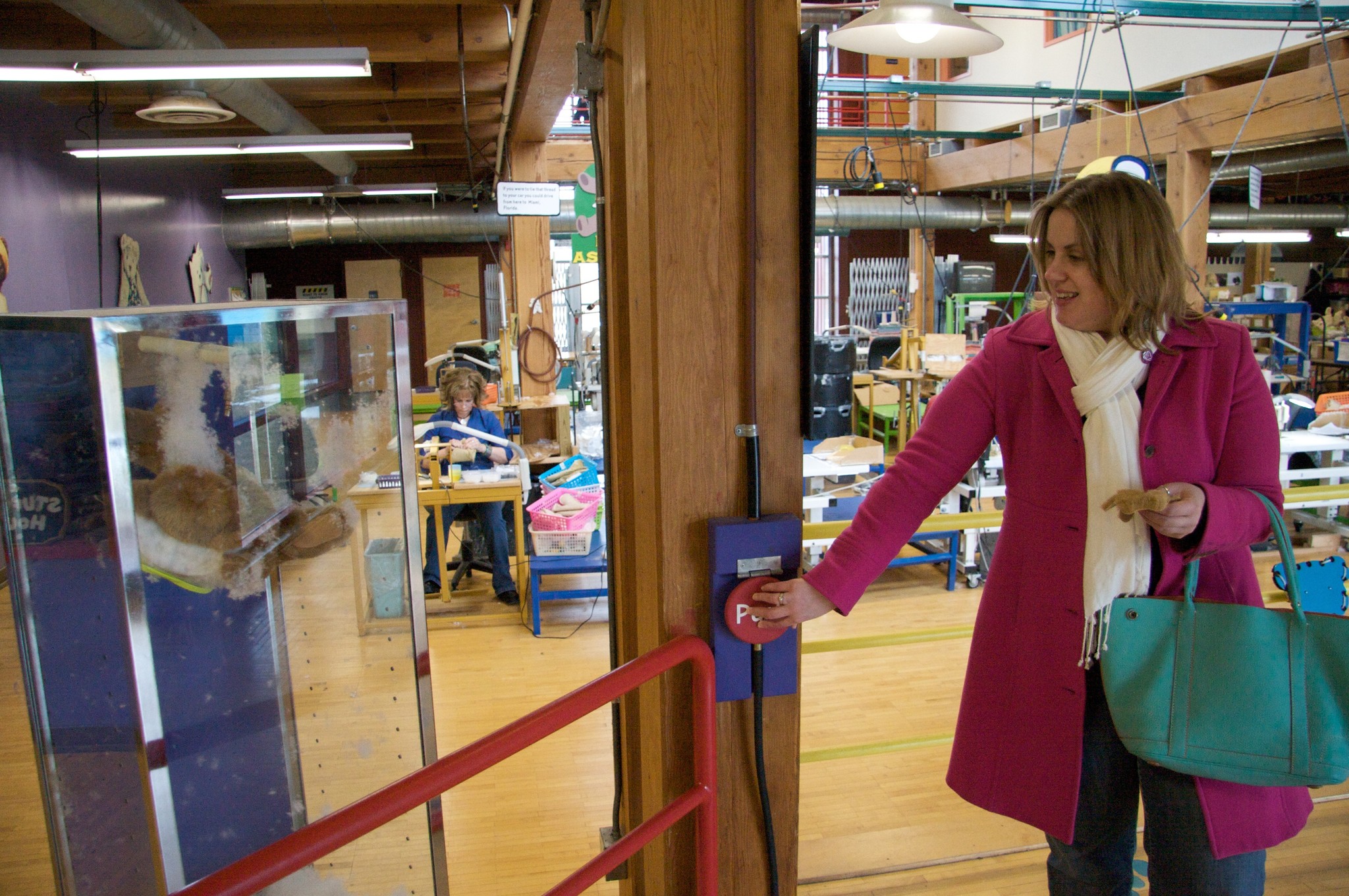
[
  {"left": 779, "top": 593, "right": 783, "bottom": 604},
  {"left": 1159, "top": 485, "right": 1170, "bottom": 495}
]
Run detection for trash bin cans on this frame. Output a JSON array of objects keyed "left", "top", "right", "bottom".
[{"left": 364, "top": 537, "right": 405, "bottom": 619}]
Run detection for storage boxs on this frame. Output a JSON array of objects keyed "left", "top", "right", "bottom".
[
  {"left": 812, "top": 434, "right": 883, "bottom": 465},
  {"left": 855, "top": 382, "right": 899, "bottom": 404}
]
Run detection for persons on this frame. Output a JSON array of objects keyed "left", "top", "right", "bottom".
[
  {"left": 746, "top": 171, "right": 1314, "bottom": 896},
  {"left": 422, "top": 366, "right": 519, "bottom": 606}
]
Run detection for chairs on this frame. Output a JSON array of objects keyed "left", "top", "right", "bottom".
[
  {"left": 867, "top": 336, "right": 900, "bottom": 371},
  {"left": 424, "top": 505, "right": 493, "bottom": 587}
]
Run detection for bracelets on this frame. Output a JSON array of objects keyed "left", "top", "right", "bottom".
[{"left": 482, "top": 443, "right": 492, "bottom": 457}]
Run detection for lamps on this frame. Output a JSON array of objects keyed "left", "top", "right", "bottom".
[
  {"left": 827, "top": 1, "right": 1003, "bottom": 57},
  {"left": 0, "top": 46, "right": 372, "bottom": 82},
  {"left": 1205, "top": 228, "right": 1312, "bottom": 243},
  {"left": 63, "top": 132, "right": 415, "bottom": 159},
  {"left": 221, "top": 183, "right": 439, "bottom": 200}
]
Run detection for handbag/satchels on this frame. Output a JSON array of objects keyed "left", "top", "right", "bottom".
[{"left": 1099, "top": 490, "right": 1349, "bottom": 786}]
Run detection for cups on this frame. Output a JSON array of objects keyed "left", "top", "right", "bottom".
[{"left": 448, "top": 464, "right": 461, "bottom": 482}]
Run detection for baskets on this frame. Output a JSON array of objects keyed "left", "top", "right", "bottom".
[
  {"left": 375, "top": 474, "right": 401, "bottom": 489},
  {"left": 528, "top": 453, "right": 605, "bottom": 556}
]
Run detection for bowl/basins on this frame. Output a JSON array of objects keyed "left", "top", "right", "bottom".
[{"left": 461, "top": 469, "right": 501, "bottom": 483}]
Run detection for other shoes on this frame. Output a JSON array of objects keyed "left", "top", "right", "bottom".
[
  {"left": 497, "top": 589, "right": 520, "bottom": 605},
  {"left": 423, "top": 579, "right": 441, "bottom": 595}
]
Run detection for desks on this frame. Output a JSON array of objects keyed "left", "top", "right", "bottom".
[
  {"left": 802, "top": 366, "right": 1348, "bottom": 588},
  {"left": 530, "top": 520, "right": 608, "bottom": 635},
  {"left": 346, "top": 463, "right": 528, "bottom": 634}
]
